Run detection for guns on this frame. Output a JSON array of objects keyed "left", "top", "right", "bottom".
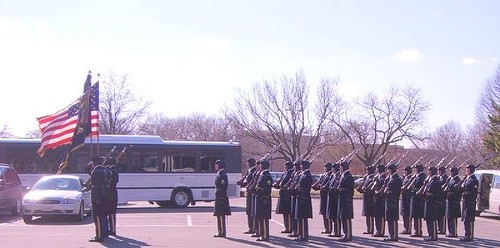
[
  {"left": 237, "top": 143, "right": 281, "bottom": 191},
  {"left": 272, "top": 146, "right": 324, "bottom": 191},
  {"left": 312, "top": 146, "right": 363, "bottom": 192},
  {"left": 82, "top": 145, "right": 127, "bottom": 192},
  {"left": 400, "top": 153, "right": 496, "bottom": 195},
  {"left": 355, "top": 149, "right": 410, "bottom": 196}
]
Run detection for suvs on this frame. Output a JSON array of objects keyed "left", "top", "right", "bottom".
[{"left": 0, "top": 163, "right": 24, "bottom": 217}]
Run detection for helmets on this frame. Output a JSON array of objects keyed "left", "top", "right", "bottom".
[
  {"left": 93, "top": 155, "right": 116, "bottom": 165},
  {"left": 216, "top": 160, "right": 225, "bottom": 169},
  {"left": 340, "top": 162, "right": 349, "bottom": 173},
  {"left": 300, "top": 161, "right": 310, "bottom": 171},
  {"left": 255, "top": 159, "right": 270, "bottom": 169},
  {"left": 284, "top": 161, "right": 293, "bottom": 170},
  {"left": 365, "top": 164, "right": 475, "bottom": 176},
  {"left": 331, "top": 164, "right": 340, "bottom": 174},
  {"left": 292, "top": 160, "right": 301, "bottom": 171},
  {"left": 324, "top": 162, "right": 332, "bottom": 172},
  {"left": 247, "top": 157, "right": 256, "bottom": 167}
]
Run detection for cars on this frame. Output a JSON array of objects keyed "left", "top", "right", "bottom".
[
  {"left": 269, "top": 171, "right": 284, "bottom": 182},
  {"left": 21, "top": 175, "right": 92, "bottom": 224},
  {"left": 352, "top": 174, "right": 364, "bottom": 187},
  {"left": 311, "top": 174, "right": 321, "bottom": 182}
]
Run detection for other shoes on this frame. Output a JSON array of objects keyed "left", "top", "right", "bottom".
[
  {"left": 251, "top": 233, "right": 260, "bottom": 237},
  {"left": 327, "top": 234, "right": 340, "bottom": 237},
  {"left": 362, "top": 232, "right": 474, "bottom": 241},
  {"left": 294, "top": 238, "right": 306, "bottom": 241},
  {"left": 280, "top": 229, "right": 292, "bottom": 233},
  {"left": 244, "top": 231, "right": 253, "bottom": 234},
  {"left": 213, "top": 233, "right": 224, "bottom": 237},
  {"left": 321, "top": 231, "right": 331, "bottom": 234},
  {"left": 88, "top": 230, "right": 111, "bottom": 241},
  {"left": 256, "top": 238, "right": 266, "bottom": 241},
  {"left": 338, "top": 238, "right": 351, "bottom": 242},
  {"left": 287, "top": 233, "right": 299, "bottom": 237}
]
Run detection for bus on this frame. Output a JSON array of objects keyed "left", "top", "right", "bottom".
[{"left": 0, "top": 134, "right": 243, "bottom": 208}]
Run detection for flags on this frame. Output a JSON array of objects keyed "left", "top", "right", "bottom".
[
  {"left": 38, "top": 82, "right": 99, "bottom": 158},
  {"left": 56, "top": 75, "right": 92, "bottom": 174}
]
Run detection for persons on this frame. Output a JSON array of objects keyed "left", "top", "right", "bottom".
[
  {"left": 400, "top": 164, "right": 478, "bottom": 242},
  {"left": 357, "top": 164, "right": 402, "bottom": 242},
  {"left": 320, "top": 162, "right": 354, "bottom": 242},
  {"left": 275, "top": 160, "right": 313, "bottom": 241},
  {"left": 213, "top": 160, "right": 231, "bottom": 237},
  {"left": 482, "top": 176, "right": 491, "bottom": 192},
  {"left": 86, "top": 155, "right": 119, "bottom": 242},
  {"left": 244, "top": 157, "right": 273, "bottom": 241}
]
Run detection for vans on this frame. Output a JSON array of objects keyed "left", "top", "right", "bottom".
[{"left": 467, "top": 169, "right": 500, "bottom": 217}]
